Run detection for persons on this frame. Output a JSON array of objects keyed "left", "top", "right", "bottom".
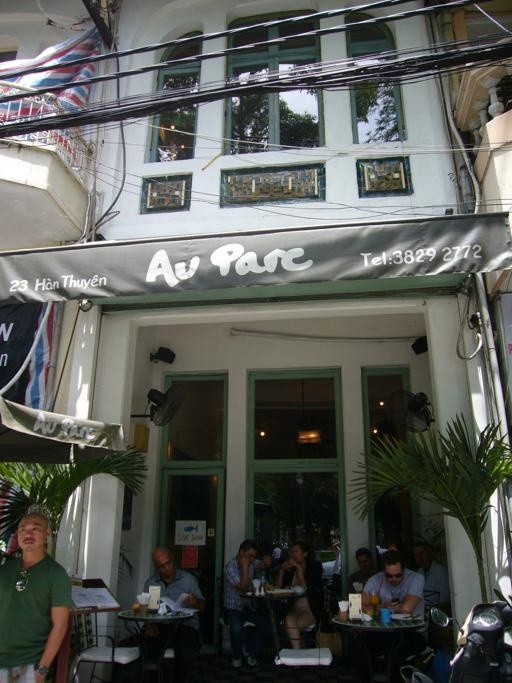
[
  {"left": 356, "top": 549, "right": 425, "bottom": 682},
  {"left": 222, "top": 538, "right": 266, "bottom": 668},
  {"left": 409, "top": 542, "right": 452, "bottom": 626},
  {"left": 268, "top": 536, "right": 281, "bottom": 560},
  {"left": 347, "top": 543, "right": 381, "bottom": 591},
  {"left": 1, "top": 510, "right": 73, "bottom": 682},
  {"left": 329, "top": 536, "right": 341, "bottom": 615},
  {"left": 280, "top": 538, "right": 323, "bottom": 664},
  {"left": 140, "top": 545, "right": 206, "bottom": 681}
]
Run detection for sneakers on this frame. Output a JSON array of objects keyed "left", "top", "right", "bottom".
[{"left": 232, "top": 644, "right": 258, "bottom": 667}]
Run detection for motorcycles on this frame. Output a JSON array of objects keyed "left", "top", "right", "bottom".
[{"left": 429, "top": 588, "right": 511, "bottom": 682}]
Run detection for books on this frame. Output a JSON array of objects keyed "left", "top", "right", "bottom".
[
  {"left": 68, "top": 584, "right": 120, "bottom": 610},
  {"left": 160, "top": 594, "right": 200, "bottom": 617}
]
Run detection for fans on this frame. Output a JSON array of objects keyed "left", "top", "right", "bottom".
[
  {"left": 131, "top": 384, "right": 184, "bottom": 426},
  {"left": 384, "top": 390, "right": 435, "bottom": 433}
]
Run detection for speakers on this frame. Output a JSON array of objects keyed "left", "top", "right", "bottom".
[
  {"left": 412, "top": 336, "right": 427, "bottom": 355},
  {"left": 156, "top": 347, "right": 175, "bottom": 363}
]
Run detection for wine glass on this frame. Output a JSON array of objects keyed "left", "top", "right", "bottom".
[{"left": 368, "top": 588, "right": 381, "bottom": 620}]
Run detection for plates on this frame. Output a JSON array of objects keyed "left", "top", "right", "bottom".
[{"left": 389, "top": 613, "right": 423, "bottom": 621}]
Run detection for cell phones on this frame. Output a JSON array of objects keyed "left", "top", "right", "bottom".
[{"left": 392, "top": 590, "right": 400, "bottom": 602}]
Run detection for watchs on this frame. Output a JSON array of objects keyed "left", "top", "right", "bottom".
[{"left": 34, "top": 662, "right": 51, "bottom": 677}]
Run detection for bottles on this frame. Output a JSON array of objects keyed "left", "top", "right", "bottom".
[
  {"left": 261, "top": 587, "right": 266, "bottom": 597},
  {"left": 255, "top": 587, "right": 260, "bottom": 595},
  {"left": 157, "top": 601, "right": 169, "bottom": 614}
]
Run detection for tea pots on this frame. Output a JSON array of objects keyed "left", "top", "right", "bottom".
[{"left": 290, "top": 585, "right": 308, "bottom": 595}]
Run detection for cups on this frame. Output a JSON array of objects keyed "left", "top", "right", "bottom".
[
  {"left": 252, "top": 579, "right": 261, "bottom": 592},
  {"left": 338, "top": 611, "right": 348, "bottom": 622},
  {"left": 139, "top": 604, "right": 148, "bottom": 614},
  {"left": 380, "top": 608, "right": 392, "bottom": 623}
]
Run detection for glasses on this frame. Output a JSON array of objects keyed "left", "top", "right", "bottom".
[{"left": 16, "top": 567, "right": 29, "bottom": 592}]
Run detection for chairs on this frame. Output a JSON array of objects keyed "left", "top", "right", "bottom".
[
  {"left": 213, "top": 575, "right": 231, "bottom": 663},
  {"left": 70, "top": 606, "right": 140, "bottom": 683},
  {"left": 265, "top": 596, "right": 333, "bottom": 682},
  {"left": 158, "top": 619, "right": 205, "bottom": 682}
]
